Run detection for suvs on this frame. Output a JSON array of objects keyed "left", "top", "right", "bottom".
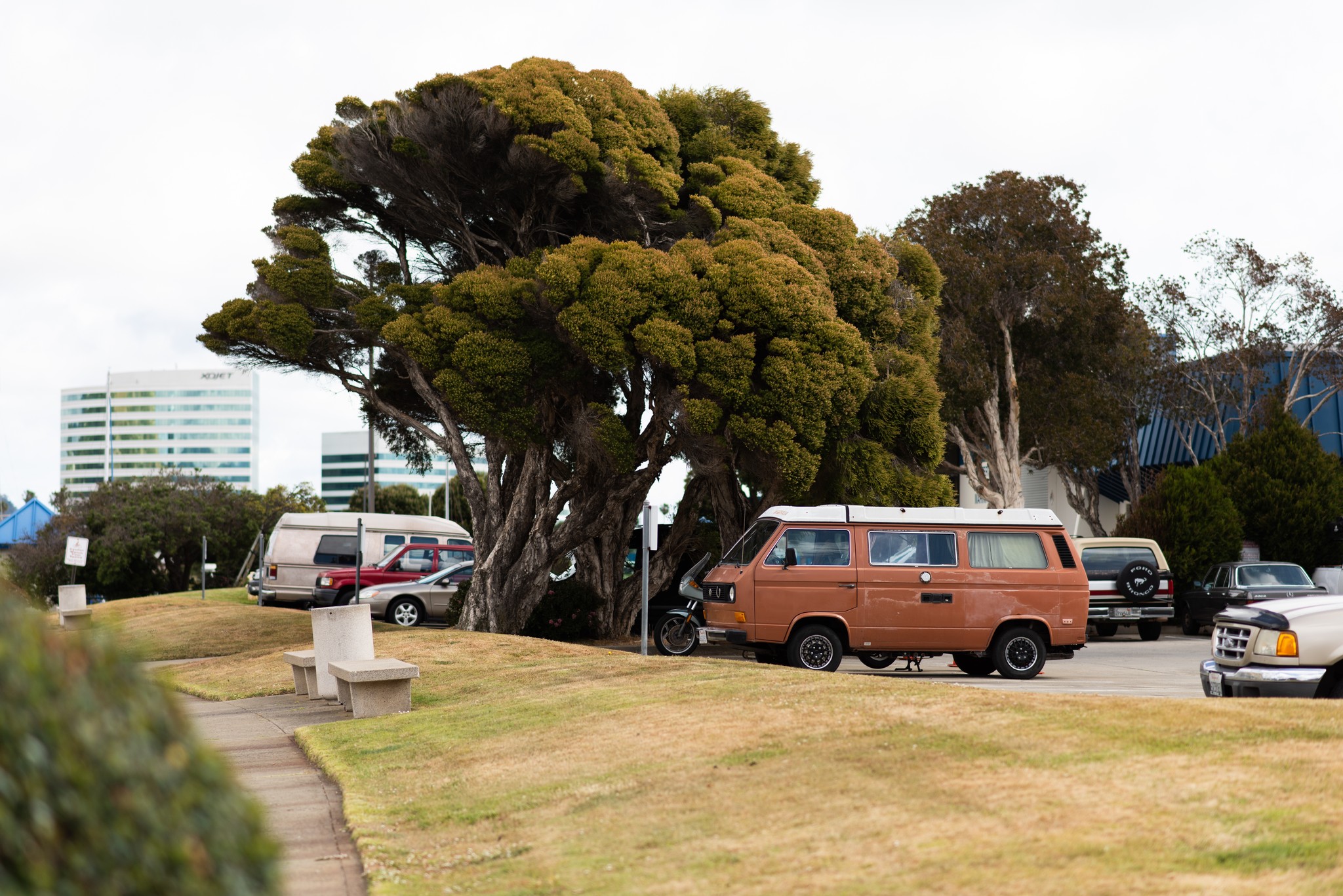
[
  {"left": 313, "top": 543, "right": 474, "bottom": 606},
  {"left": 1069, "top": 534, "right": 1175, "bottom": 641},
  {"left": 1199, "top": 595, "right": 1343, "bottom": 698}
]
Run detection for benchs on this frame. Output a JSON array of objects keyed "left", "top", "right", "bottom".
[
  {"left": 56, "top": 606, "right": 92, "bottom": 631},
  {"left": 328, "top": 658, "right": 419, "bottom": 718},
  {"left": 283, "top": 650, "right": 322, "bottom": 701}
]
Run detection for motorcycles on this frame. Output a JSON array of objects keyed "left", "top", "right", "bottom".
[{"left": 652, "top": 552, "right": 712, "bottom": 658}]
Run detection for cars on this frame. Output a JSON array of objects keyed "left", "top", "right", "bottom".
[
  {"left": 1178, "top": 561, "right": 1328, "bottom": 635},
  {"left": 348, "top": 560, "right": 474, "bottom": 628}
]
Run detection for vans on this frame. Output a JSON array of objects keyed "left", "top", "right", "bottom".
[
  {"left": 259, "top": 512, "right": 474, "bottom": 609},
  {"left": 693, "top": 502, "right": 1090, "bottom": 680}
]
